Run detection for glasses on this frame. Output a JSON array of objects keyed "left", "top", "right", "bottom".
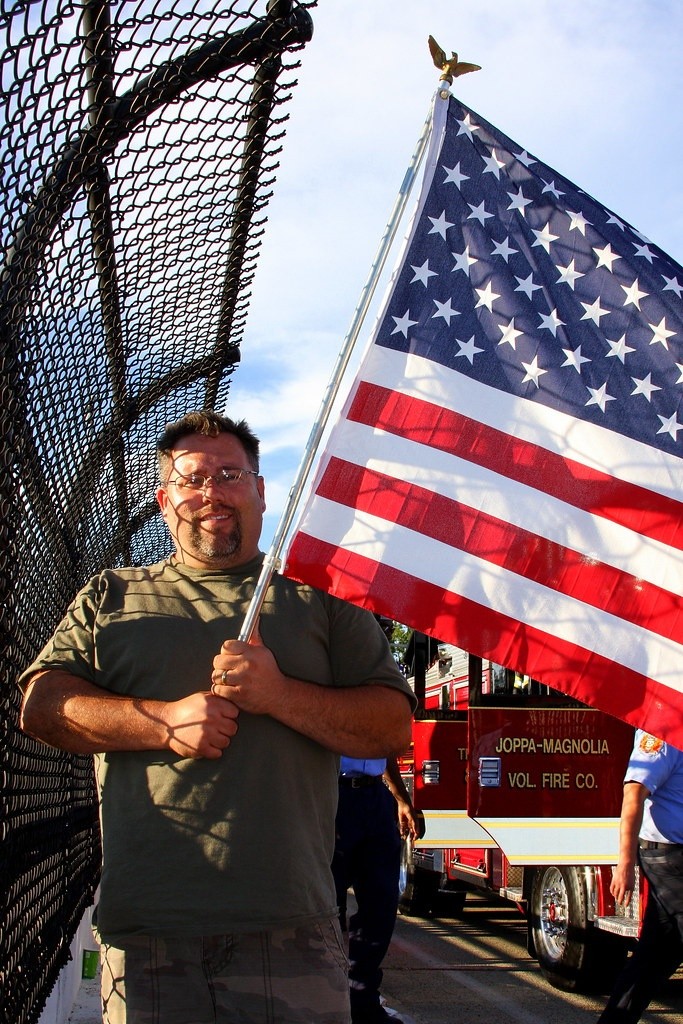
[{"left": 160, "top": 470, "right": 259, "bottom": 492}]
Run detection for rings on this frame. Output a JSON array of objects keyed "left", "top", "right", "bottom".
[{"left": 221, "top": 669, "right": 227, "bottom": 685}]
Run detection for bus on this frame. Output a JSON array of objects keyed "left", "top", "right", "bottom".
[{"left": 397, "top": 629, "right": 649, "bottom": 994}]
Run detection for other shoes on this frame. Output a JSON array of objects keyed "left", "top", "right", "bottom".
[{"left": 352, "top": 1004, "right": 403, "bottom": 1024}]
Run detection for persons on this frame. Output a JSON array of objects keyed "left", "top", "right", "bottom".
[
  {"left": 15, "top": 414, "right": 421, "bottom": 1024},
  {"left": 594, "top": 725, "right": 683, "bottom": 1024}
]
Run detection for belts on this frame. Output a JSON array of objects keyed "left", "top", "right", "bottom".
[
  {"left": 338, "top": 776, "right": 382, "bottom": 789},
  {"left": 642, "top": 839, "right": 683, "bottom": 850}
]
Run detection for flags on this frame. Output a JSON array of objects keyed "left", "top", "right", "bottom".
[{"left": 278, "top": 83, "right": 683, "bottom": 751}]
